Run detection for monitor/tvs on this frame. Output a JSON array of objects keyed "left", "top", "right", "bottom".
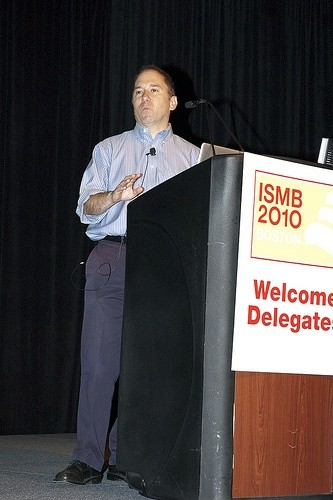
[{"left": 197, "top": 142, "right": 244, "bottom": 162}]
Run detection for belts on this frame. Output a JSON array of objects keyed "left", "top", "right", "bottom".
[{"left": 102, "top": 235, "right": 127, "bottom": 244}]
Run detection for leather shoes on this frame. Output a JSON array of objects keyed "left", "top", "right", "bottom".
[
  {"left": 106, "top": 464, "right": 128, "bottom": 481},
  {"left": 53, "top": 460, "right": 104, "bottom": 484}
]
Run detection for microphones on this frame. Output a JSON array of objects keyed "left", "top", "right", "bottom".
[
  {"left": 185, "top": 99, "right": 247, "bottom": 153},
  {"left": 150, "top": 148, "right": 155, "bottom": 156}
]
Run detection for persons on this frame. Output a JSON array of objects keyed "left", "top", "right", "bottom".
[{"left": 53, "top": 64, "right": 200, "bottom": 486}]
